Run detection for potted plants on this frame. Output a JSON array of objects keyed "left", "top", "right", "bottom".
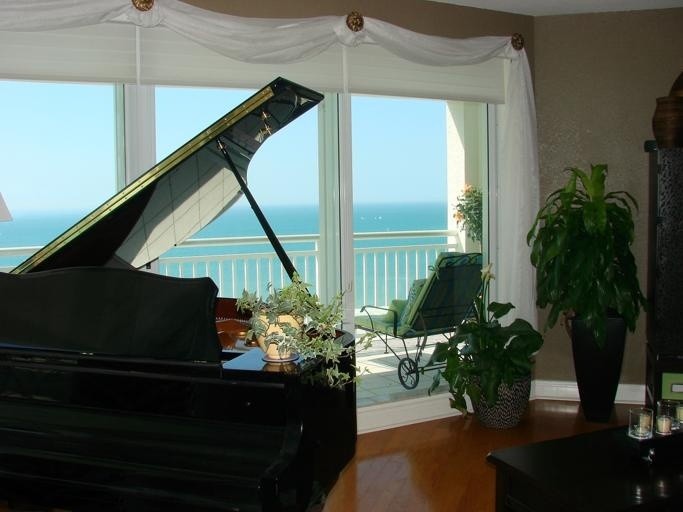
[{"left": 236, "top": 270, "right": 378, "bottom": 391}]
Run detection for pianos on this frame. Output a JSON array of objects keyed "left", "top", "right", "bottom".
[{"left": 0, "top": 77, "right": 356, "bottom": 512}]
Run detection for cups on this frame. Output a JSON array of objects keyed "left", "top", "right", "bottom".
[
  {"left": 676, "top": 406, "right": 683, "bottom": 424},
  {"left": 656, "top": 400, "right": 679, "bottom": 430},
  {"left": 628, "top": 408, "right": 653, "bottom": 438},
  {"left": 655, "top": 414, "right": 672, "bottom": 433}
]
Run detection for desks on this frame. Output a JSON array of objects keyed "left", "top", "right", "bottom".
[{"left": 485, "top": 414, "right": 683, "bottom": 512}]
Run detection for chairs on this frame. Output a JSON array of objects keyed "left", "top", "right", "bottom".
[{"left": 351, "top": 249, "right": 484, "bottom": 390}]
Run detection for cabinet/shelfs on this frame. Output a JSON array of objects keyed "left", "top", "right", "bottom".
[{"left": 644, "top": 140, "right": 683, "bottom": 433}]
